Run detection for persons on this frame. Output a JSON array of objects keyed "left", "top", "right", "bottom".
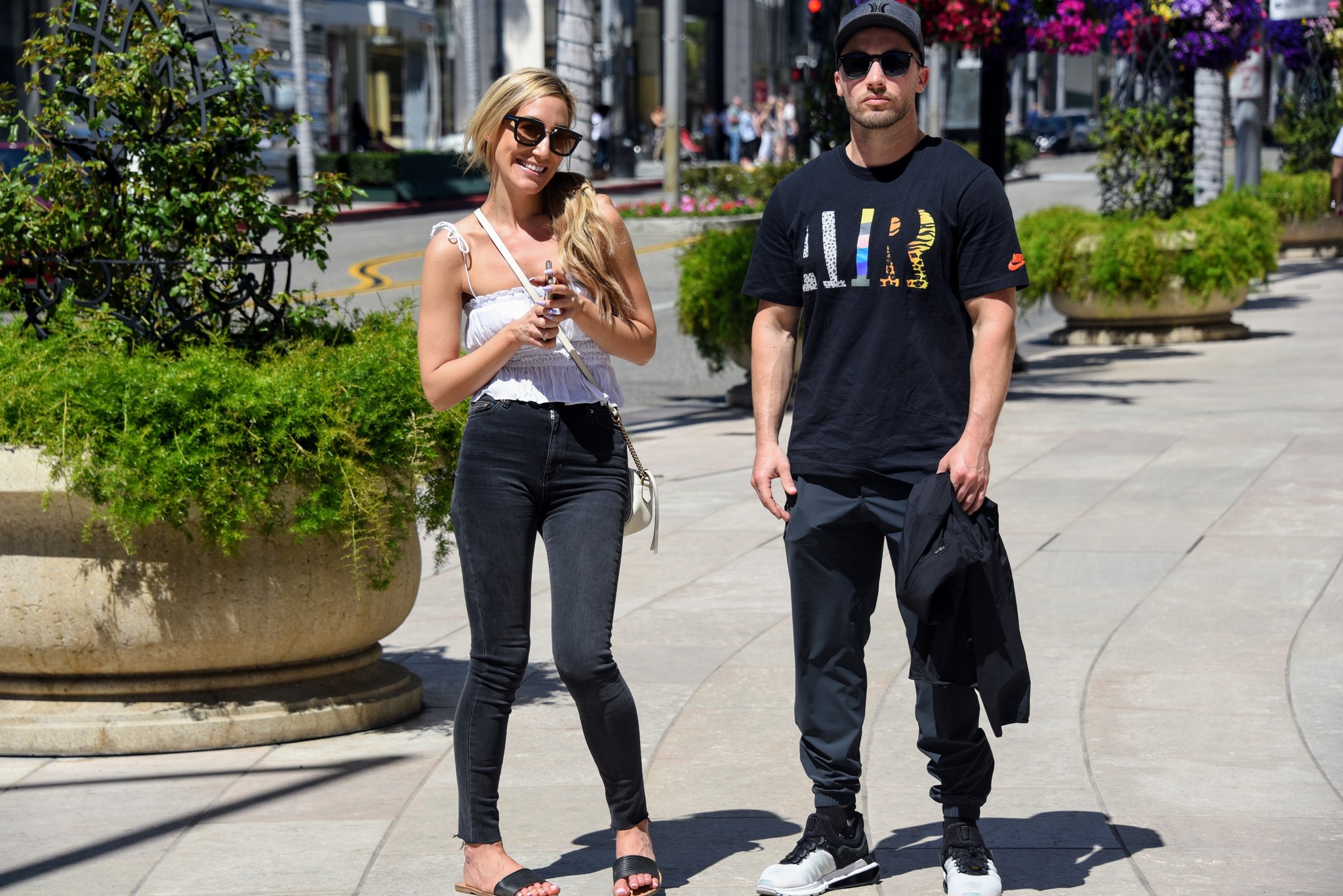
[
  {"left": 1330, "top": 125, "right": 1343, "bottom": 218},
  {"left": 742, "top": 0, "right": 1031, "bottom": 896},
  {"left": 698, "top": 81, "right": 820, "bottom": 172},
  {"left": 650, "top": 103, "right": 665, "bottom": 163},
  {"left": 417, "top": 66, "right": 662, "bottom": 896}
]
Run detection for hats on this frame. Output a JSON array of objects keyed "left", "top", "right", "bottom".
[{"left": 834, "top": 0, "right": 926, "bottom": 66}]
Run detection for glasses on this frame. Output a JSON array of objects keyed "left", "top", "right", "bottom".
[
  {"left": 505, "top": 113, "right": 583, "bottom": 156},
  {"left": 838, "top": 49, "right": 923, "bottom": 80}
]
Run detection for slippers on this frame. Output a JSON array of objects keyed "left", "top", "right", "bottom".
[
  {"left": 612, "top": 854, "right": 662, "bottom": 896},
  {"left": 455, "top": 868, "right": 561, "bottom": 896}
]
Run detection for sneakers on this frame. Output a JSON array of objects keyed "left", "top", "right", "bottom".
[
  {"left": 938, "top": 820, "right": 1003, "bottom": 896},
  {"left": 756, "top": 811, "right": 880, "bottom": 896}
]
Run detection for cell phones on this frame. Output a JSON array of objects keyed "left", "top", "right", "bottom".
[{"left": 542, "top": 260, "right": 554, "bottom": 342}]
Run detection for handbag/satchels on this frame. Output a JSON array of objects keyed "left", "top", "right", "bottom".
[{"left": 623, "top": 468, "right": 659, "bottom": 554}]
